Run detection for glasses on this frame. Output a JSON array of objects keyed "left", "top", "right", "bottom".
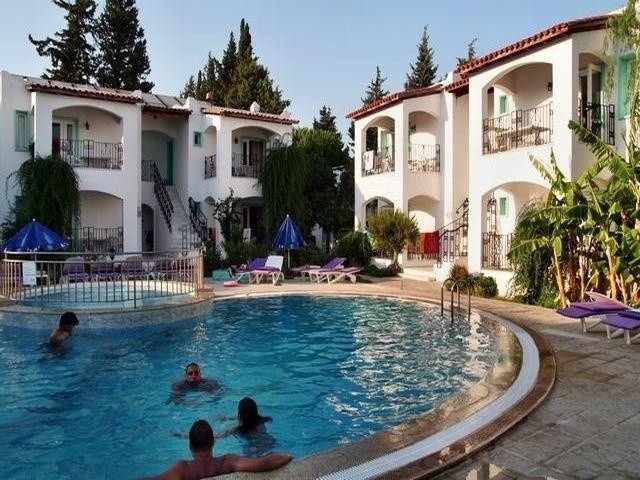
[{"left": 185, "top": 372, "right": 199, "bottom": 375}]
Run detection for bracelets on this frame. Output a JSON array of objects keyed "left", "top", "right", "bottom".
[{"left": 288, "top": 452, "right": 292, "bottom": 460}]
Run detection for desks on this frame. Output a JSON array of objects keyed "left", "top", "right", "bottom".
[{"left": 497, "top": 124, "right": 550, "bottom": 149}]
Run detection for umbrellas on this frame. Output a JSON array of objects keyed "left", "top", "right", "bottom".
[
  {"left": 272, "top": 214, "right": 308, "bottom": 269},
  {"left": 0, "top": 219, "right": 70, "bottom": 261}
]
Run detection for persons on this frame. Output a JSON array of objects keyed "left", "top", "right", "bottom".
[
  {"left": 213, "top": 396, "right": 272, "bottom": 439},
  {"left": 173, "top": 362, "right": 222, "bottom": 404},
  {"left": 134, "top": 419, "right": 294, "bottom": 479},
  {"left": 49, "top": 311, "right": 80, "bottom": 341}
]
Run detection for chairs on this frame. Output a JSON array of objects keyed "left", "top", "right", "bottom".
[
  {"left": 555, "top": 292, "right": 640, "bottom": 346},
  {"left": 234, "top": 253, "right": 368, "bottom": 286},
  {"left": 58, "top": 255, "right": 193, "bottom": 285}
]
